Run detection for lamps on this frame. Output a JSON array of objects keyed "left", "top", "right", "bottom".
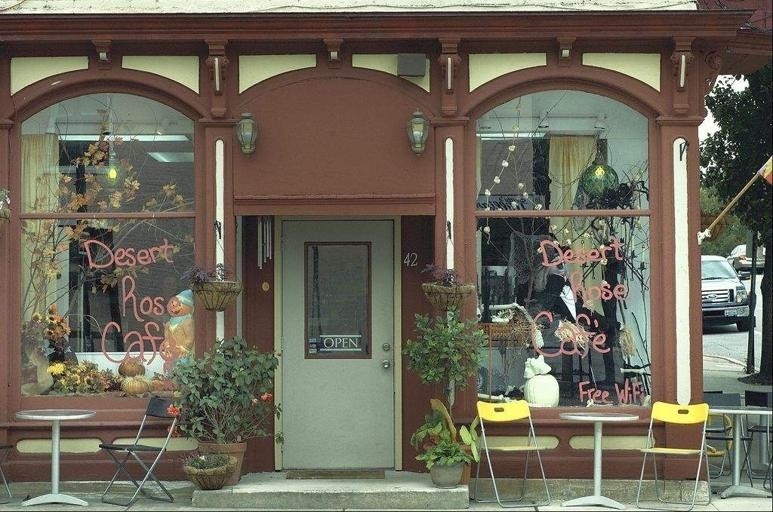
[
  {"left": 405, "top": 108, "right": 433, "bottom": 159},
  {"left": 233, "top": 113, "right": 258, "bottom": 156}
]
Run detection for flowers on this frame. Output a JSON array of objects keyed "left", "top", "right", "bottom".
[
  {"left": 21, "top": 304, "right": 120, "bottom": 396},
  {"left": 159, "top": 334, "right": 286, "bottom": 470}
]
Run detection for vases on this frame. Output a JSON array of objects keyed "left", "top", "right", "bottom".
[{"left": 183, "top": 463, "right": 235, "bottom": 491}]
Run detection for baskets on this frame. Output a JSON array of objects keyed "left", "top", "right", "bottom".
[{"left": 479, "top": 323, "right": 522, "bottom": 347}]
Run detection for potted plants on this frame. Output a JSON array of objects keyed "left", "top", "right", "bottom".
[
  {"left": 179, "top": 219, "right": 243, "bottom": 313},
  {"left": 400, "top": 261, "right": 478, "bottom": 497}
]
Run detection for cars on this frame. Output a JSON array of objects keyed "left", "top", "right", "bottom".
[
  {"left": 700, "top": 255, "right": 753, "bottom": 332},
  {"left": 724, "top": 244, "right": 766, "bottom": 273}
]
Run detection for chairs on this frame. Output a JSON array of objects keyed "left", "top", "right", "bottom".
[
  {"left": 740, "top": 424, "right": 773, "bottom": 487},
  {"left": 95, "top": 394, "right": 184, "bottom": 509},
  {"left": 635, "top": 400, "right": 712, "bottom": 510},
  {"left": 706, "top": 414, "right": 733, "bottom": 478},
  {"left": 472, "top": 399, "right": 550, "bottom": 508}
]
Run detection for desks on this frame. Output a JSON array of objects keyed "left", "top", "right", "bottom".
[
  {"left": 709, "top": 408, "right": 772, "bottom": 499},
  {"left": 12, "top": 407, "right": 94, "bottom": 507},
  {"left": 557, "top": 410, "right": 639, "bottom": 510}
]
